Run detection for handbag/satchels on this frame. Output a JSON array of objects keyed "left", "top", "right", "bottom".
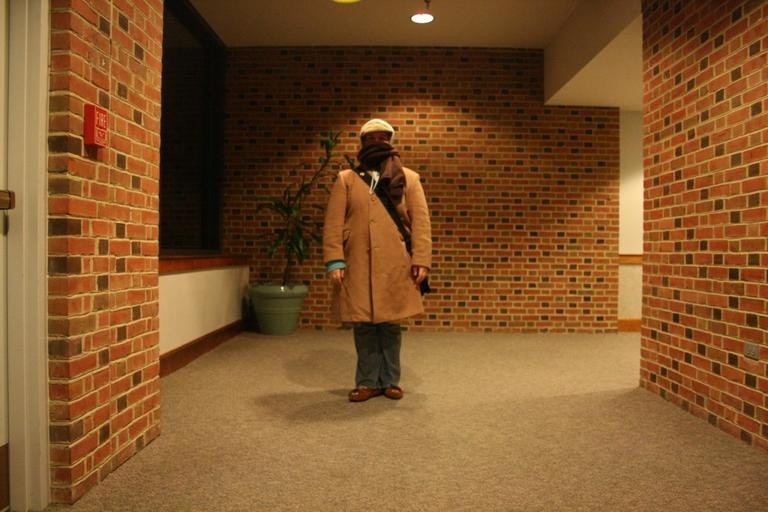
[{"left": 419, "top": 278, "right": 431, "bottom": 296}]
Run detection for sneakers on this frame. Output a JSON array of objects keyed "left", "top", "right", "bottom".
[
  {"left": 384, "top": 386, "right": 404, "bottom": 400},
  {"left": 348, "top": 386, "right": 384, "bottom": 402}
]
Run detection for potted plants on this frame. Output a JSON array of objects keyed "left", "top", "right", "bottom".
[{"left": 245, "top": 128, "right": 356, "bottom": 335}]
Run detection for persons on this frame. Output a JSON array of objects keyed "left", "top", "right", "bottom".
[{"left": 323, "top": 118, "right": 433, "bottom": 402}]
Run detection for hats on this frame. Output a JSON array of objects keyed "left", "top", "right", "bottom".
[{"left": 360, "top": 117, "right": 395, "bottom": 140}]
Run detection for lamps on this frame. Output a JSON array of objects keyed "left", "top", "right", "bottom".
[{"left": 410, "top": 9, "right": 434, "bottom": 24}]
